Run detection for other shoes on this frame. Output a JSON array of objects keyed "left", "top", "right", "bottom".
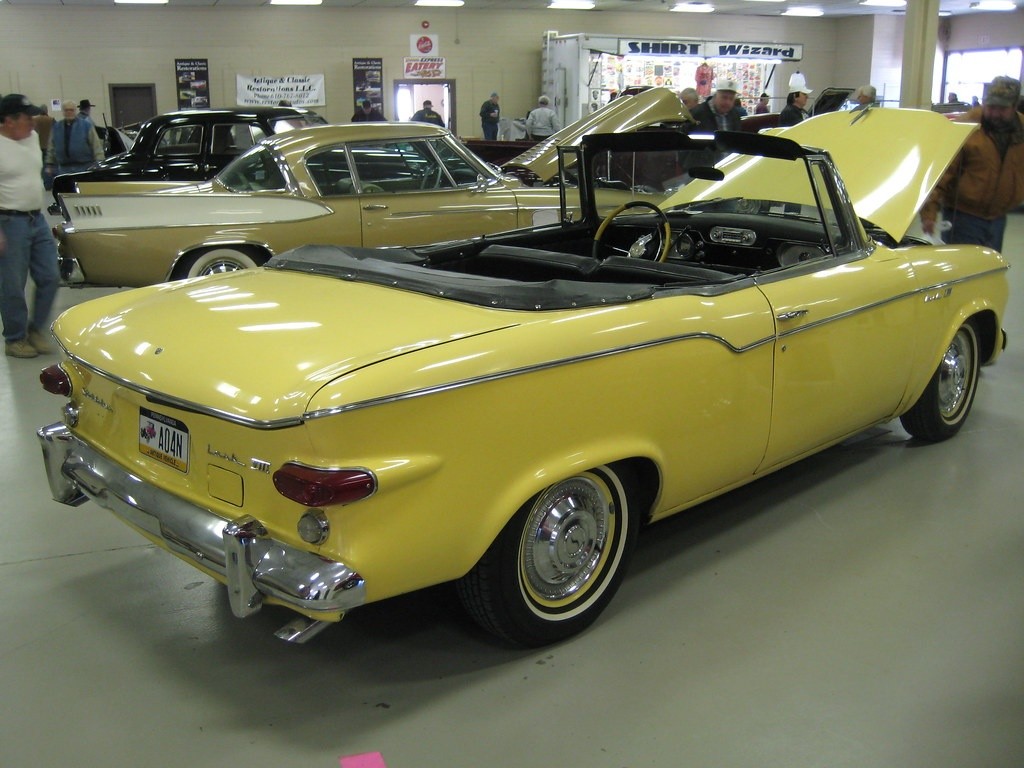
[
  {"left": 5, "top": 339, "right": 37, "bottom": 357},
  {"left": 27, "top": 330, "right": 54, "bottom": 353}
]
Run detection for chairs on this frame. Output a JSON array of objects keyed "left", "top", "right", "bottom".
[
  {"left": 337, "top": 178, "right": 384, "bottom": 193},
  {"left": 598, "top": 257, "right": 735, "bottom": 285}
]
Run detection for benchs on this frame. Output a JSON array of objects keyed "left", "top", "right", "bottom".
[{"left": 480, "top": 244, "right": 599, "bottom": 283}]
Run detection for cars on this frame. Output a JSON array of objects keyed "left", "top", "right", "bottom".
[{"left": 46, "top": 105, "right": 501, "bottom": 220}]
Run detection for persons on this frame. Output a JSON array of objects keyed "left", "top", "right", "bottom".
[
  {"left": 480, "top": 93, "right": 500, "bottom": 140},
  {"left": 857, "top": 85, "right": 876, "bottom": 104},
  {"left": 351, "top": 101, "right": 388, "bottom": 122},
  {"left": 34, "top": 104, "right": 56, "bottom": 151},
  {"left": 591, "top": 90, "right": 599, "bottom": 111},
  {"left": 411, "top": 100, "right": 445, "bottom": 127},
  {"left": 691, "top": 81, "right": 748, "bottom": 132},
  {"left": 77, "top": 99, "right": 96, "bottom": 127},
  {"left": 0, "top": 94, "right": 61, "bottom": 358},
  {"left": 948, "top": 93, "right": 959, "bottom": 103},
  {"left": 526, "top": 96, "right": 560, "bottom": 140},
  {"left": 47, "top": 100, "right": 104, "bottom": 177},
  {"left": 608, "top": 92, "right": 617, "bottom": 103},
  {"left": 972, "top": 96, "right": 979, "bottom": 106},
  {"left": 757, "top": 93, "right": 769, "bottom": 113},
  {"left": 779, "top": 83, "right": 813, "bottom": 126},
  {"left": 279, "top": 100, "right": 292, "bottom": 107},
  {"left": 920, "top": 76, "right": 1024, "bottom": 252},
  {"left": 680, "top": 87, "right": 697, "bottom": 111}
]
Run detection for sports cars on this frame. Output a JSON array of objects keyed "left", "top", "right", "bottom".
[
  {"left": 34, "top": 97, "right": 1011, "bottom": 655},
  {"left": 53, "top": 89, "right": 708, "bottom": 296}
]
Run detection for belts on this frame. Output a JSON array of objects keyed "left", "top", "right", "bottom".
[{"left": 0, "top": 210, "right": 41, "bottom": 215}]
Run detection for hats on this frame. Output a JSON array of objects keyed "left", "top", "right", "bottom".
[
  {"left": 855, "top": 85, "right": 876, "bottom": 99},
  {"left": 280, "top": 101, "right": 291, "bottom": 107},
  {"left": 77, "top": 100, "right": 94, "bottom": 107},
  {"left": 362, "top": 101, "right": 371, "bottom": 108},
  {"left": 0, "top": 94, "right": 41, "bottom": 116},
  {"left": 986, "top": 76, "right": 1020, "bottom": 106},
  {"left": 423, "top": 100, "right": 433, "bottom": 108},
  {"left": 790, "top": 85, "right": 813, "bottom": 94},
  {"left": 716, "top": 77, "right": 739, "bottom": 94}
]
